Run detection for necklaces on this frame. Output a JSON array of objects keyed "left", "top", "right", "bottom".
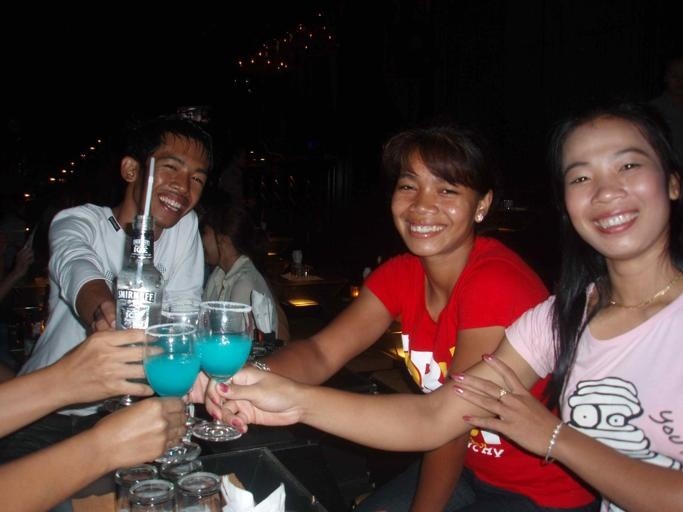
[{"left": 602, "top": 271, "right": 681, "bottom": 311}]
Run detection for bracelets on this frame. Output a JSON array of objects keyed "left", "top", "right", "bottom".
[
  {"left": 541, "top": 420, "right": 569, "bottom": 464},
  {"left": 252, "top": 358, "right": 272, "bottom": 372}
]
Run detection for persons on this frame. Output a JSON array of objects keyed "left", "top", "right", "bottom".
[
  {"left": 11, "top": 106, "right": 218, "bottom": 384},
  {"left": 0, "top": 394, "right": 191, "bottom": 511},
  {"left": 200, "top": 90, "right": 682, "bottom": 511},
  {"left": 176, "top": 121, "right": 600, "bottom": 512},
  {"left": 211, "top": 141, "right": 258, "bottom": 210},
  {"left": 0, "top": 324, "right": 169, "bottom": 444},
  {"left": 0, "top": 224, "right": 38, "bottom": 305},
  {"left": 197, "top": 186, "right": 278, "bottom": 300},
  {"left": 198, "top": 200, "right": 294, "bottom": 358}
]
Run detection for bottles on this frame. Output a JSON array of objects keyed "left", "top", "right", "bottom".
[{"left": 111, "top": 212, "right": 164, "bottom": 406}]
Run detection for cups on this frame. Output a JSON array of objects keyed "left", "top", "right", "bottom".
[
  {"left": 112, "top": 464, "right": 159, "bottom": 512},
  {"left": 127, "top": 479, "right": 175, "bottom": 511},
  {"left": 158, "top": 456, "right": 206, "bottom": 481},
  {"left": 176, "top": 471, "right": 222, "bottom": 512}
]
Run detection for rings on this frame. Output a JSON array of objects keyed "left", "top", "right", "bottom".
[{"left": 497, "top": 389, "right": 509, "bottom": 400}]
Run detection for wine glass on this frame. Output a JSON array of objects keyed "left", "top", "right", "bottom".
[
  {"left": 160, "top": 302, "right": 198, "bottom": 439},
  {"left": 190, "top": 300, "right": 253, "bottom": 443},
  {"left": 140, "top": 322, "right": 201, "bottom": 467}
]
